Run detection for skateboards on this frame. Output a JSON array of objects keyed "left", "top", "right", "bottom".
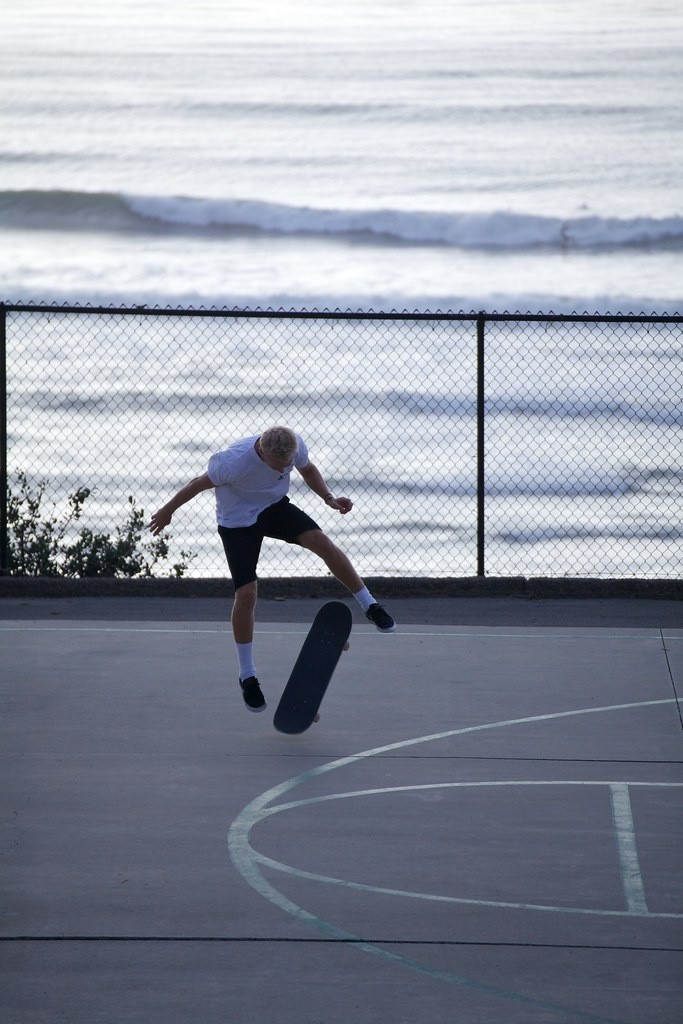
[{"left": 271, "top": 601, "right": 355, "bottom": 736}]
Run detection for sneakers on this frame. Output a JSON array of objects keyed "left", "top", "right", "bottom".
[
  {"left": 238, "top": 675, "right": 266, "bottom": 713},
  {"left": 366, "top": 602, "right": 397, "bottom": 633}
]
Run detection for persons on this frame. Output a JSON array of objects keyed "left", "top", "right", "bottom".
[{"left": 149, "top": 424, "right": 396, "bottom": 713}]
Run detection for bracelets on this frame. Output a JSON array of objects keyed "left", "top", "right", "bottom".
[{"left": 323, "top": 493, "right": 334, "bottom": 502}]
